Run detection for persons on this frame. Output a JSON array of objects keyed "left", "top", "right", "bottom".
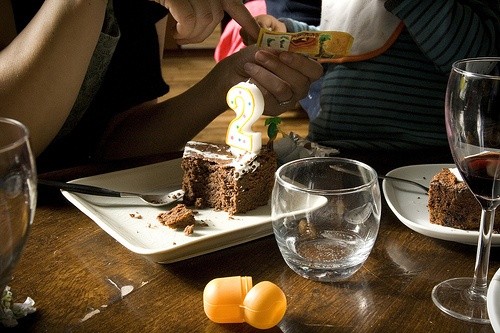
[
  {"left": 0, "top": 0, "right": 323, "bottom": 175},
  {"left": 240, "top": 1, "right": 500, "bottom": 155}
]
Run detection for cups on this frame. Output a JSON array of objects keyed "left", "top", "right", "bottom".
[{"left": 271, "top": 157, "right": 382, "bottom": 283}]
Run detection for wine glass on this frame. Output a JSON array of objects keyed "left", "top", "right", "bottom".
[
  {"left": 430, "top": 55, "right": 500, "bottom": 324},
  {"left": 1, "top": 116, "right": 36, "bottom": 332}
]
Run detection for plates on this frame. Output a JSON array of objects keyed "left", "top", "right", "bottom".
[
  {"left": 486, "top": 266, "right": 500, "bottom": 333},
  {"left": 60, "top": 157, "right": 328, "bottom": 266},
  {"left": 383, "top": 163, "right": 500, "bottom": 247}
]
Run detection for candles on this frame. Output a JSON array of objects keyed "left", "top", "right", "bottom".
[{"left": 225, "top": 77, "right": 268, "bottom": 152}]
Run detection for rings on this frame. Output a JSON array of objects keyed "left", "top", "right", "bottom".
[{"left": 279, "top": 95, "right": 295, "bottom": 105}]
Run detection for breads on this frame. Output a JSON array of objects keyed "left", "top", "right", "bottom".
[
  {"left": 157, "top": 139, "right": 279, "bottom": 235},
  {"left": 427, "top": 167, "right": 500, "bottom": 231}
]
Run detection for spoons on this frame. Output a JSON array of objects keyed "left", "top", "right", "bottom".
[{"left": 37, "top": 178, "right": 186, "bottom": 207}]
